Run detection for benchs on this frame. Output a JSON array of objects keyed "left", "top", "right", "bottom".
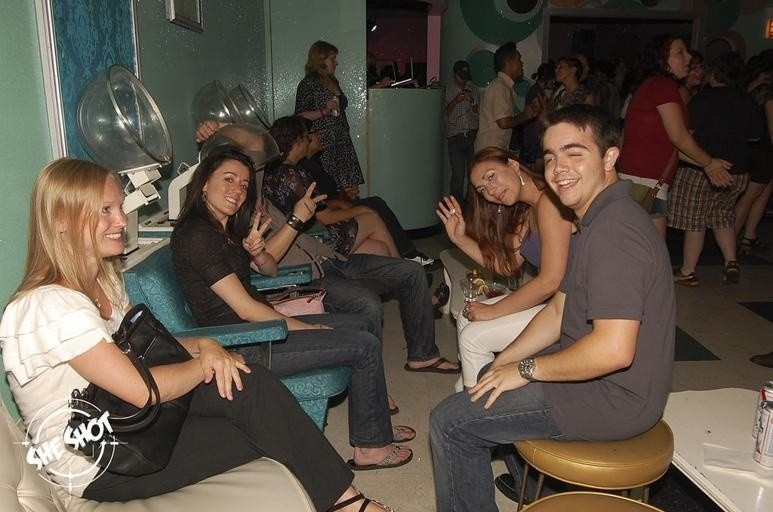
[{"left": 439, "top": 247, "right": 518, "bottom": 320}]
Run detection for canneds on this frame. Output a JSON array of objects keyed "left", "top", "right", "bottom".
[{"left": 752, "top": 380, "right": 773, "bottom": 469}]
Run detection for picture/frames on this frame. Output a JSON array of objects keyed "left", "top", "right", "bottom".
[
  {"left": 33, "top": 0, "right": 146, "bottom": 183},
  {"left": 163, "top": 0, "right": 205, "bottom": 34}
]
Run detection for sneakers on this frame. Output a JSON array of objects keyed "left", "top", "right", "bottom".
[
  {"left": 721, "top": 259, "right": 741, "bottom": 285},
  {"left": 402, "top": 252, "right": 444, "bottom": 272},
  {"left": 672, "top": 266, "right": 701, "bottom": 289},
  {"left": 737, "top": 237, "right": 773, "bottom": 257}
]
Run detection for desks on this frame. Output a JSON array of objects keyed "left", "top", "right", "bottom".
[{"left": 654, "top": 382, "right": 772, "bottom": 512}]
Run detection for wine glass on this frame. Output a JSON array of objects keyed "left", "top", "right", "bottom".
[{"left": 459, "top": 268, "right": 503, "bottom": 303}]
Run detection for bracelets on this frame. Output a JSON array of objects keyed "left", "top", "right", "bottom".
[
  {"left": 287, "top": 215, "right": 304, "bottom": 232},
  {"left": 320, "top": 108, "right": 325, "bottom": 117}
]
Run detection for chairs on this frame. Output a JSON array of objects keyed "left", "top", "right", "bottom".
[{"left": 0, "top": 183, "right": 353, "bottom": 512}]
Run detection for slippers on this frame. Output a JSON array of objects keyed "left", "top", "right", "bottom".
[
  {"left": 391, "top": 424, "right": 417, "bottom": 443},
  {"left": 346, "top": 443, "right": 415, "bottom": 473},
  {"left": 403, "top": 356, "right": 464, "bottom": 376},
  {"left": 430, "top": 282, "right": 451, "bottom": 308}
]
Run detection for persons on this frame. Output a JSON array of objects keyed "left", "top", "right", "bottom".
[
  {"left": 0, "top": 158, "right": 400, "bottom": 512},
  {"left": 293, "top": 39, "right": 366, "bottom": 188},
  {"left": 368, "top": 49, "right": 420, "bottom": 88},
  {"left": 196, "top": 119, "right": 462, "bottom": 415},
  {"left": 169, "top": 147, "right": 417, "bottom": 469},
  {"left": 304, "top": 117, "right": 441, "bottom": 272},
  {"left": 263, "top": 115, "right": 450, "bottom": 319}
]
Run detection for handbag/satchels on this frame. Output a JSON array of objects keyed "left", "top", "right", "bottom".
[
  {"left": 618, "top": 146, "right": 680, "bottom": 217},
  {"left": 61, "top": 301, "right": 201, "bottom": 478},
  {"left": 267, "top": 287, "right": 329, "bottom": 318}
]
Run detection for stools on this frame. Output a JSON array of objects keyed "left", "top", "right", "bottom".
[
  {"left": 515, "top": 487, "right": 663, "bottom": 511},
  {"left": 515, "top": 420, "right": 675, "bottom": 504}
]
